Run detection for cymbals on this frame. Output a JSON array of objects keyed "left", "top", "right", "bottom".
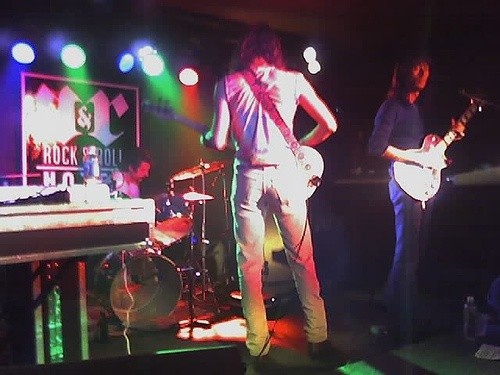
[
  {"left": 178, "top": 189, "right": 215, "bottom": 201},
  {"left": 169, "top": 160, "right": 224, "bottom": 180}
]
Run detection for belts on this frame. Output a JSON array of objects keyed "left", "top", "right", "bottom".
[{"left": 236, "top": 164, "right": 280, "bottom": 169}]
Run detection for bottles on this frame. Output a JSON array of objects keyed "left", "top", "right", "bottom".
[
  {"left": 463, "top": 297, "right": 478, "bottom": 341},
  {"left": 82, "top": 146, "right": 99, "bottom": 185}
]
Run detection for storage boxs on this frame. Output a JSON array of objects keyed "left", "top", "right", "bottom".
[{"left": 0, "top": 198, "right": 154, "bottom": 266}]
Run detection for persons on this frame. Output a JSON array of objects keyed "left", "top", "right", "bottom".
[
  {"left": 367, "top": 49, "right": 465, "bottom": 349},
  {"left": 200, "top": 30, "right": 341, "bottom": 364},
  {"left": 105, "top": 147, "right": 151, "bottom": 199}
]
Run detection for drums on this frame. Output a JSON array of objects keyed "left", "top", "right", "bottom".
[
  {"left": 94, "top": 248, "right": 183, "bottom": 326},
  {"left": 150, "top": 194, "right": 191, "bottom": 240}
]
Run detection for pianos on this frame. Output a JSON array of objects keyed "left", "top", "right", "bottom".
[{"left": 1, "top": 181, "right": 155, "bottom": 267}]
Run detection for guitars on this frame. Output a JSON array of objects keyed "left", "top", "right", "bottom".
[
  {"left": 389, "top": 99, "right": 482, "bottom": 202},
  {"left": 141, "top": 98, "right": 323, "bottom": 199}
]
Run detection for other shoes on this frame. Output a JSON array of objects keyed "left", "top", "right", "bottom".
[{"left": 307, "top": 340, "right": 327, "bottom": 354}]
